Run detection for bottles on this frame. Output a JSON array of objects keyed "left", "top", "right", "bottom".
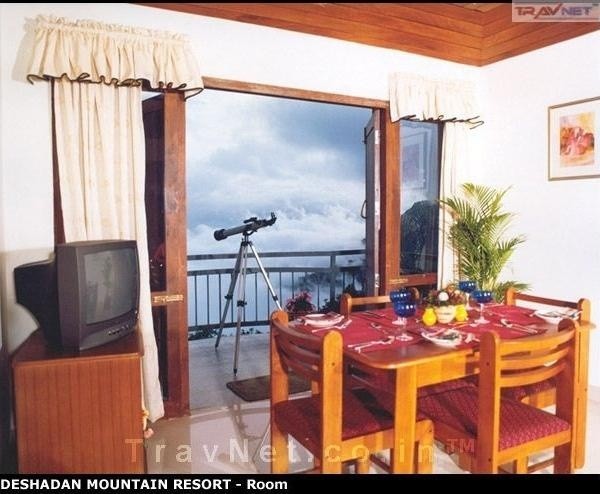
[
  {"left": 422, "top": 306, "right": 439, "bottom": 326},
  {"left": 454, "top": 304, "right": 468, "bottom": 322}
]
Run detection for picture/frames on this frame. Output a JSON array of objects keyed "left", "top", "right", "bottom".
[{"left": 546, "top": 95, "right": 599, "bottom": 180}]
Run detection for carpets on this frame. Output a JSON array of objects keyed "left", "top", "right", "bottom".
[{"left": 226, "top": 371, "right": 312, "bottom": 402}]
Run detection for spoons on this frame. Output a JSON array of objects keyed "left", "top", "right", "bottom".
[
  {"left": 347, "top": 337, "right": 392, "bottom": 348},
  {"left": 371, "top": 322, "right": 392, "bottom": 336},
  {"left": 501, "top": 318, "right": 538, "bottom": 333}
]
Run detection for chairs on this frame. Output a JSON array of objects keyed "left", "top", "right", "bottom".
[{"left": 265, "top": 282, "right": 591, "bottom": 474}]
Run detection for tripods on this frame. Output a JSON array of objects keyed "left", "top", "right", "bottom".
[{"left": 214, "top": 234, "right": 283, "bottom": 375}]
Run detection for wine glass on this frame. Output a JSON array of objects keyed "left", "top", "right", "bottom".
[
  {"left": 390, "top": 288, "right": 415, "bottom": 342},
  {"left": 459, "top": 280, "right": 493, "bottom": 323}
]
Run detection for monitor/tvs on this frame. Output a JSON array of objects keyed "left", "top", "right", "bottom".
[{"left": 13, "top": 237, "right": 140, "bottom": 352}]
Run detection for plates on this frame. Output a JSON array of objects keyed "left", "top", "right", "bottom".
[
  {"left": 304, "top": 312, "right": 341, "bottom": 327},
  {"left": 534, "top": 306, "right": 574, "bottom": 324},
  {"left": 422, "top": 331, "right": 461, "bottom": 347}
]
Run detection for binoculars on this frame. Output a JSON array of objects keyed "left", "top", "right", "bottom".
[{"left": 214, "top": 211, "right": 282, "bottom": 377}]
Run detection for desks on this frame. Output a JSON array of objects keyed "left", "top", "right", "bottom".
[{"left": 9, "top": 323, "right": 146, "bottom": 474}]
